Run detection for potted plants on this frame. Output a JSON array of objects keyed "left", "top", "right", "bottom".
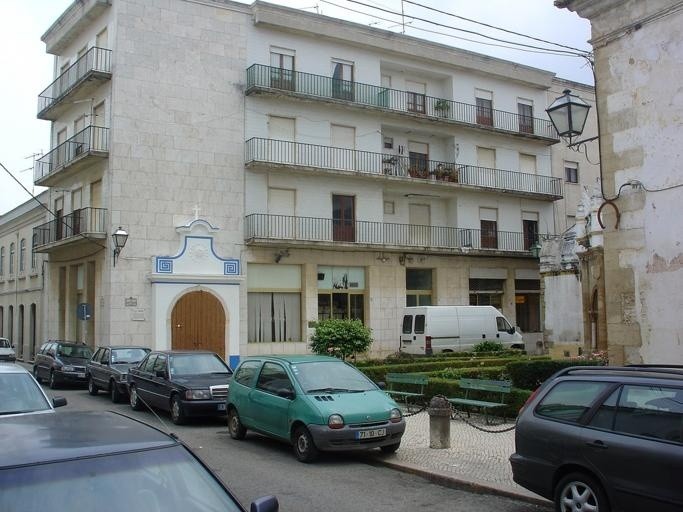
[{"left": 409, "top": 157, "right": 466, "bottom": 182}]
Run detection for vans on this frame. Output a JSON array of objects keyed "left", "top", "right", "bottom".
[{"left": 397, "top": 303, "right": 526, "bottom": 356}]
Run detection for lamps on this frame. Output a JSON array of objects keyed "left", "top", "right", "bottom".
[
  {"left": 529, "top": 239, "right": 543, "bottom": 264},
  {"left": 111, "top": 225, "right": 129, "bottom": 268},
  {"left": 544, "top": 87, "right": 599, "bottom": 148}
]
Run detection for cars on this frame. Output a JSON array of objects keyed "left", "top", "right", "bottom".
[
  {"left": 225, "top": 352, "right": 406, "bottom": 464},
  {"left": 31, "top": 338, "right": 96, "bottom": 391},
  {"left": 0, "top": 408, "right": 281, "bottom": 512},
  {"left": 1, "top": 337, "right": 17, "bottom": 364},
  {"left": 0, "top": 361, "right": 69, "bottom": 417},
  {"left": 125, "top": 348, "right": 237, "bottom": 426},
  {"left": 509, "top": 361, "right": 683, "bottom": 512},
  {"left": 84, "top": 344, "right": 156, "bottom": 405}
]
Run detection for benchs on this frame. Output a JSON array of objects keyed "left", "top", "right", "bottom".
[{"left": 380, "top": 372, "right": 515, "bottom": 427}]
